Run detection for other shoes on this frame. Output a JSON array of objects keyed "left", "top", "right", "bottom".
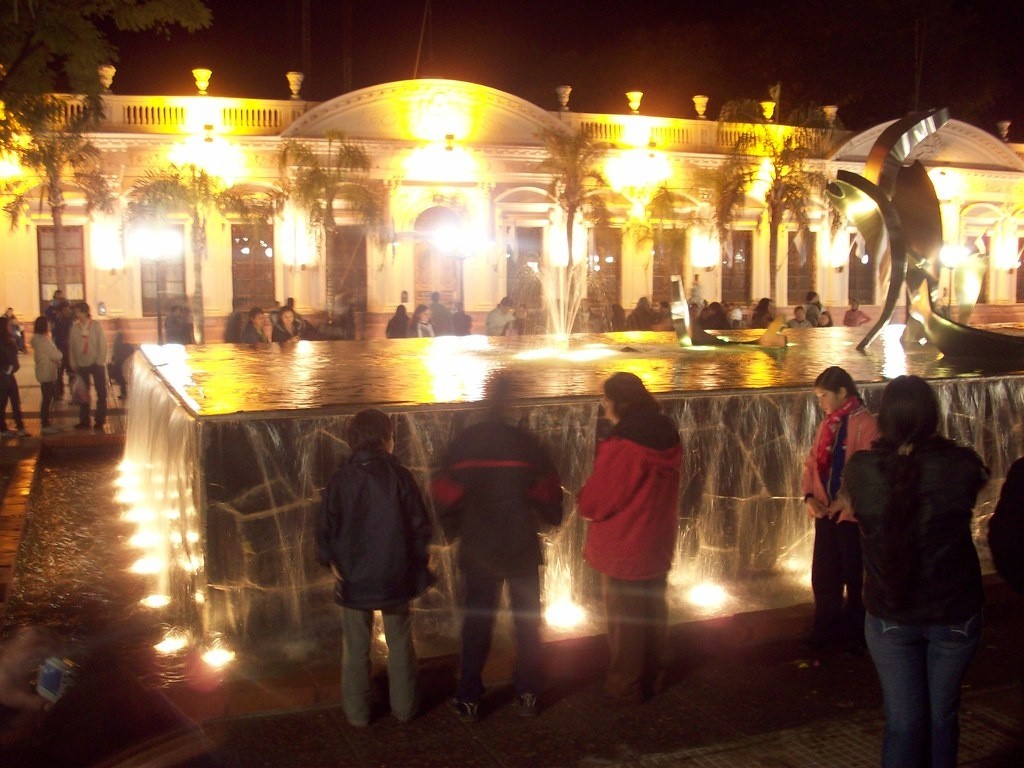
[
  {"left": 0, "top": 429, "right": 12, "bottom": 437},
  {"left": 74, "top": 423, "right": 88, "bottom": 430},
  {"left": 450, "top": 698, "right": 480, "bottom": 721},
  {"left": 42, "top": 425, "right": 60, "bottom": 434},
  {"left": 17, "top": 430, "right": 31, "bottom": 436},
  {"left": 94, "top": 422, "right": 102, "bottom": 430},
  {"left": 512, "top": 691, "right": 540, "bottom": 717}
]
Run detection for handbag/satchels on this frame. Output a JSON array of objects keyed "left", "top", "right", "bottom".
[{"left": 69, "top": 374, "right": 88, "bottom": 403}]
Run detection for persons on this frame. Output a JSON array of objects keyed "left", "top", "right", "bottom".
[
  {"left": 577, "top": 372, "right": 683, "bottom": 703},
  {"left": 224, "top": 298, "right": 323, "bottom": 343},
  {"left": 0, "top": 308, "right": 33, "bottom": 440},
  {"left": 987, "top": 457, "right": 1024, "bottom": 591},
  {"left": 799, "top": 364, "right": 882, "bottom": 659},
  {"left": 109, "top": 316, "right": 134, "bottom": 399},
  {"left": 0, "top": 624, "right": 66, "bottom": 713},
  {"left": 785, "top": 291, "right": 833, "bottom": 328},
  {"left": 317, "top": 308, "right": 347, "bottom": 340},
  {"left": 429, "top": 372, "right": 564, "bottom": 722},
  {"left": 313, "top": 409, "right": 433, "bottom": 728},
  {"left": 166, "top": 305, "right": 196, "bottom": 345},
  {"left": 627, "top": 297, "right": 778, "bottom": 331},
  {"left": 843, "top": 375, "right": 991, "bottom": 768},
  {"left": 385, "top": 292, "right": 472, "bottom": 338},
  {"left": 28, "top": 289, "right": 108, "bottom": 434},
  {"left": 843, "top": 299, "right": 872, "bottom": 327},
  {"left": 485, "top": 296, "right": 626, "bottom": 335}
]
